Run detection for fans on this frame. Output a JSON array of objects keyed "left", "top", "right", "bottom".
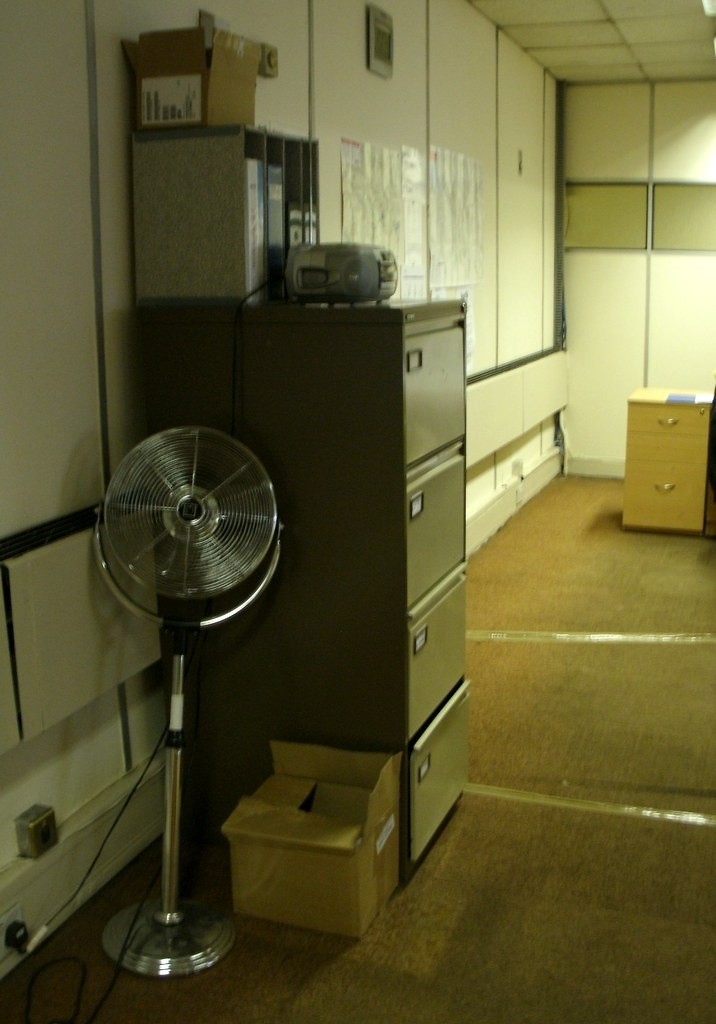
[{"left": 93, "top": 425, "right": 284, "bottom": 978}]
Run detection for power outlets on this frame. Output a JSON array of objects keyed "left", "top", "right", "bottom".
[{"left": 0, "top": 903, "right": 27, "bottom": 964}]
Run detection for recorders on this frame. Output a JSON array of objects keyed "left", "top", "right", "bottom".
[{"left": 291, "top": 137, "right": 397, "bottom": 304}]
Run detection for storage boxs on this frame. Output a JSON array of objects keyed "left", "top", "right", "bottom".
[
  {"left": 119, "top": 28, "right": 264, "bottom": 130},
  {"left": 220, "top": 738, "right": 402, "bottom": 938}
]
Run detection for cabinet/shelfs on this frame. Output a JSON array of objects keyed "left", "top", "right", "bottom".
[
  {"left": 129, "top": 127, "right": 320, "bottom": 301},
  {"left": 142, "top": 300, "right": 470, "bottom": 882},
  {"left": 622, "top": 388, "right": 714, "bottom": 536}
]
